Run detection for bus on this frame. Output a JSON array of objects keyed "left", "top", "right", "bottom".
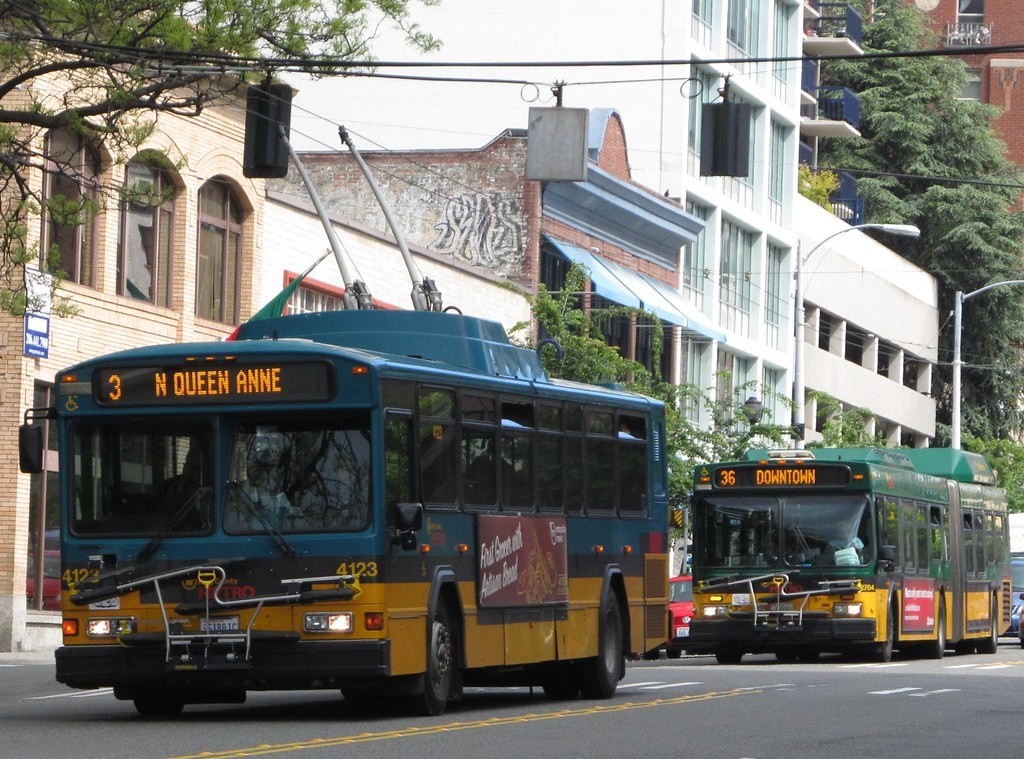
[
  {"left": 670, "top": 447, "right": 1010, "bottom": 665},
  {"left": 18, "top": 307, "right": 670, "bottom": 721}
]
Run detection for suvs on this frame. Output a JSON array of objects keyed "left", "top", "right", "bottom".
[{"left": 666, "top": 572, "right": 697, "bottom": 660}]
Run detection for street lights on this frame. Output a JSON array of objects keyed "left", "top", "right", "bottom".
[
  {"left": 793, "top": 222, "right": 921, "bottom": 448},
  {"left": 743, "top": 397, "right": 765, "bottom": 438}
]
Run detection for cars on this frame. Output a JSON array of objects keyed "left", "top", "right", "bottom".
[
  {"left": 1019, "top": 594, "right": 1024, "bottom": 649},
  {"left": 997, "top": 556, "right": 1024, "bottom": 637}
]
